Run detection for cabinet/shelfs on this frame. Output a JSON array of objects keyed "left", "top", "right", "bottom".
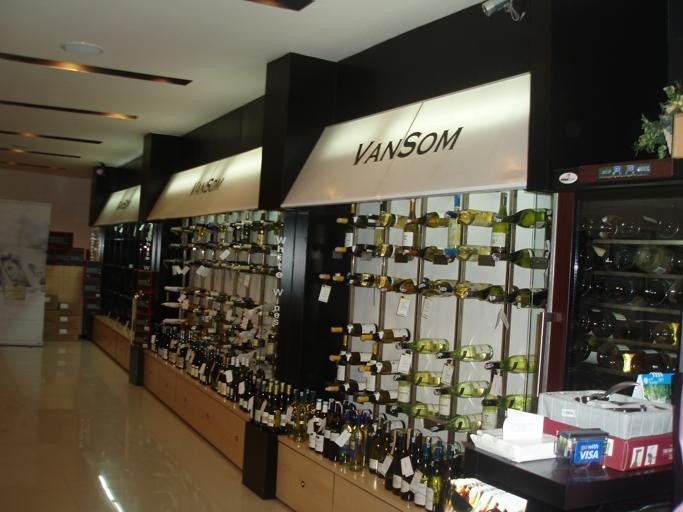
[
  {"left": 277, "top": 434, "right": 334, "bottom": 512},
  {"left": 172, "top": 365, "right": 214, "bottom": 447},
  {"left": 142, "top": 350, "right": 172, "bottom": 411},
  {"left": 334, "top": 463, "right": 426, "bottom": 512},
  {"left": 91, "top": 316, "right": 129, "bottom": 373},
  {"left": 214, "top": 392, "right": 249, "bottom": 470}
]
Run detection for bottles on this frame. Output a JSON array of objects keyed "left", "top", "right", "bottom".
[
  {"left": 141, "top": 318, "right": 290, "bottom": 434},
  {"left": 166, "top": 210, "right": 281, "bottom": 324},
  {"left": 291, "top": 380, "right": 533, "bottom": 512},
  {"left": 569, "top": 213, "right": 682, "bottom": 377},
  {"left": 318, "top": 207, "right": 550, "bottom": 310},
  {"left": 326, "top": 323, "right": 538, "bottom": 374}
]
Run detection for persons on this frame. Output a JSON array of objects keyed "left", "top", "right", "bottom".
[{"left": 0, "top": 253, "right": 45, "bottom": 287}]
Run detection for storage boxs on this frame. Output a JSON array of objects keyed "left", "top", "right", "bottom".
[{"left": 44, "top": 292, "right": 79, "bottom": 341}]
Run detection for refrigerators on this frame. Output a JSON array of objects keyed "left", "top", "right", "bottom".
[{"left": 540, "top": 156, "right": 683, "bottom": 394}]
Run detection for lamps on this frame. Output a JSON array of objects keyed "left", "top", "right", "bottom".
[{"left": 62, "top": 43, "right": 102, "bottom": 58}]
